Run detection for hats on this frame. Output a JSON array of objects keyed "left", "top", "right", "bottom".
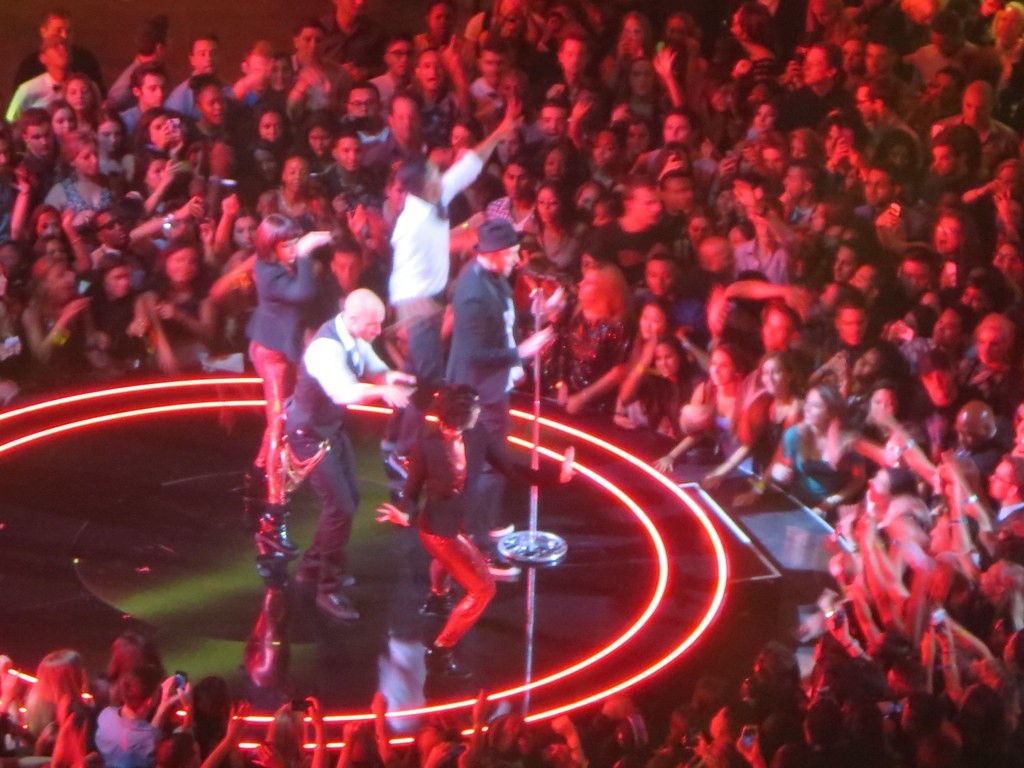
[{"left": 474, "top": 217, "right": 527, "bottom": 252}]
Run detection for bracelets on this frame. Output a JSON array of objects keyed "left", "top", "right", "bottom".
[
  {"left": 900, "top": 439, "right": 915, "bottom": 455},
  {"left": 755, "top": 482, "right": 767, "bottom": 492},
  {"left": 164, "top": 211, "right": 178, "bottom": 228},
  {"left": 959, "top": 629, "right": 971, "bottom": 639},
  {"left": 942, "top": 650, "right": 954, "bottom": 654},
  {"left": 948, "top": 518, "right": 966, "bottom": 527},
  {"left": 963, "top": 495, "right": 977, "bottom": 505},
  {"left": 844, "top": 638, "right": 856, "bottom": 650}
]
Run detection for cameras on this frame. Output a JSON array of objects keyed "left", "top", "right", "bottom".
[
  {"left": 291, "top": 698, "right": 314, "bottom": 712},
  {"left": 834, "top": 610, "right": 846, "bottom": 629},
  {"left": 898, "top": 326, "right": 915, "bottom": 342}
]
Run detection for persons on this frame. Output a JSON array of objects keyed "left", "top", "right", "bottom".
[
  {"left": 0, "top": 0, "right": 1023, "bottom": 768},
  {"left": 225, "top": 556, "right": 428, "bottom": 733}
]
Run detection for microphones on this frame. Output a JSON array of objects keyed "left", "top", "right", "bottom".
[{"left": 522, "top": 265, "right": 545, "bottom": 283}]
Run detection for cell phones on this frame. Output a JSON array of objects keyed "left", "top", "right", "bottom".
[
  {"left": 933, "top": 608, "right": 945, "bottom": 634},
  {"left": 890, "top": 203, "right": 901, "bottom": 217},
  {"left": 175, "top": 671, "right": 188, "bottom": 691},
  {"left": 168, "top": 118, "right": 181, "bottom": 137},
  {"left": 741, "top": 726, "right": 756, "bottom": 748}
]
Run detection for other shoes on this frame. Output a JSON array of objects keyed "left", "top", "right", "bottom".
[
  {"left": 294, "top": 564, "right": 357, "bottom": 588},
  {"left": 483, "top": 517, "right": 516, "bottom": 541},
  {"left": 315, "top": 591, "right": 361, "bottom": 620},
  {"left": 485, "top": 555, "right": 522, "bottom": 580}
]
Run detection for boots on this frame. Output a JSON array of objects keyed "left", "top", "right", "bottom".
[
  {"left": 254, "top": 503, "right": 301, "bottom": 561},
  {"left": 242, "top": 466, "right": 271, "bottom": 500}
]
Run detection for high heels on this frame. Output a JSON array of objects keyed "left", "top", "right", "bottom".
[
  {"left": 418, "top": 595, "right": 456, "bottom": 620},
  {"left": 423, "top": 647, "right": 474, "bottom": 684}
]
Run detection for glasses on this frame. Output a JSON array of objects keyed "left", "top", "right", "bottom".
[
  {"left": 346, "top": 99, "right": 382, "bottom": 107},
  {"left": 936, "top": 226, "right": 970, "bottom": 235},
  {"left": 96, "top": 215, "right": 125, "bottom": 231}
]
